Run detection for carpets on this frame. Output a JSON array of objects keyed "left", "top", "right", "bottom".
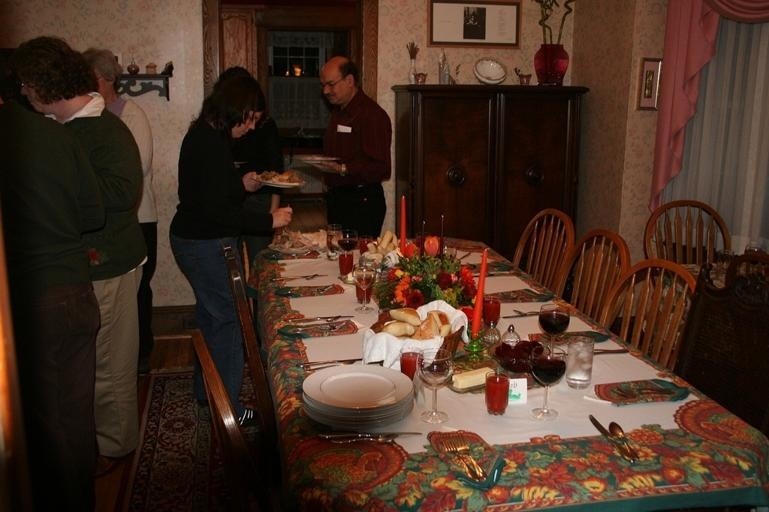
[{"left": 114, "top": 370, "right": 271, "bottom": 512}]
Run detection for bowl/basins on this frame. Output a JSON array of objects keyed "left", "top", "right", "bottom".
[{"left": 473, "top": 58, "right": 508, "bottom": 85}]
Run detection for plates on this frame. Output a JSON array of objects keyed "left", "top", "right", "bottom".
[
  {"left": 299, "top": 364, "right": 415, "bottom": 430},
  {"left": 292, "top": 156, "right": 342, "bottom": 164},
  {"left": 268, "top": 242, "right": 319, "bottom": 256},
  {"left": 249, "top": 174, "right": 306, "bottom": 188}
]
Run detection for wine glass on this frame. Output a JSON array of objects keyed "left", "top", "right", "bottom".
[
  {"left": 352, "top": 262, "right": 376, "bottom": 315},
  {"left": 538, "top": 304, "right": 571, "bottom": 354},
  {"left": 416, "top": 348, "right": 454, "bottom": 424},
  {"left": 529, "top": 346, "right": 567, "bottom": 418}
]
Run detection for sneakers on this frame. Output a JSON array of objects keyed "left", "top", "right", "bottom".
[{"left": 238, "top": 410, "right": 259, "bottom": 426}]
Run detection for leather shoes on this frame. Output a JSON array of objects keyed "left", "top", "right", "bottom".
[{"left": 94, "top": 452, "right": 135, "bottom": 477}]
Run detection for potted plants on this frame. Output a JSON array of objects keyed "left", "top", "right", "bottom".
[{"left": 406, "top": 42, "right": 421, "bottom": 82}]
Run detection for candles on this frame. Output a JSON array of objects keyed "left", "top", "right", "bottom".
[
  {"left": 398, "top": 193, "right": 410, "bottom": 246},
  {"left": 472, "top": 247, "right": 491, "bottom": 333}
]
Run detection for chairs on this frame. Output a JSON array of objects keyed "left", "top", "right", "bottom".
[{"left": 190, "top": 242, "right": 283, "bottom": 512}]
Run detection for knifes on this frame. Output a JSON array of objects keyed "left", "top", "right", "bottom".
[
  {"left": 503, "top": 314, "right": 539, "bottom": 318},
  {"left": 319, "top": 431, "right": 423, "bottom": 437},
  {"left": 589, "top": 414, "right": 632, "bottom": 464},
  {"left": 297, "top": 358, "right": 363, "bottom": 367},
  {"left": 292, "top": 315, "right": 355, "bottom": 322}
]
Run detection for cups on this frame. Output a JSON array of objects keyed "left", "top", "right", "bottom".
[
  {"left": 355, "top": 277, "right": 373, "bottom": 304},
  {"left": 566, "top": 336, "right": 595, "bottom": 389},
  {"left": 486, "top": 371, "right": 509, "bottom": 416},
  {"left": 337, "top": 248, "right": 354, "bottom": 277},
  {"left": 338, "top": 230, "right": 359, "bottom": 252},
  {"left": 326, "top": 224, "right": 343, "bottom": 260},
  {"left": 400, "top": 348, "right": 423, "bottom": 380},
  {"left": 483, "top": 296, "right": 500, "bottom": 328}
]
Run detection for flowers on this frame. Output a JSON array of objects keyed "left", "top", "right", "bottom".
[{"left": 372, "top": 232, "right": 480, "bottom": 319}]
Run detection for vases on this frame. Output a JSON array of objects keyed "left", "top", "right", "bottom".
[{"left": 533, "top": 44, "right": 570, "bottom": 86}]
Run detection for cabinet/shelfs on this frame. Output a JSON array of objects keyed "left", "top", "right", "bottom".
[{"left": 391, "top": 84, "right": 590, "bottom": 260}]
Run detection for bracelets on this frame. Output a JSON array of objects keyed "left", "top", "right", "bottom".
[{"left": 340, "top": 162, "right": 346, "bottom": 178}]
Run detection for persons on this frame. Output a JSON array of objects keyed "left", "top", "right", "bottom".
[
  {"left": 79, "top": 48, "right": 160, "bottom": 375},
  {"left": 305, "top": 51, "right": 393, "bottom": 242},
  {"left": 166, "top": 63, "right": 296, "bottom": 431},
  {"left": 220, "top": 107, "right": 286, "bottom": 266},
  {"left": 0, "top": 48, "right": 107, "bottom": 512},
  {"left": 11, "top": 32, "right": 149, "bottom": 481}
]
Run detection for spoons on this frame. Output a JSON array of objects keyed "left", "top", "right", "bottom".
[
  {"left": 512, "top": 308, "right": 538, "bottom": 316},
  {"left": 331, "top": 437, "right": 396, "bottom": 445},
  {"left": 306, "top": 361, "right": 355, "bottom": 371},
  {"left": 295, "top": 316, "right": 341, "bottom": 324},
  {"left": 608, "top": 422, "right": 640, "bottom": 462}
]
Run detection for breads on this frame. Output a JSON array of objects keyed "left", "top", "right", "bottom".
[
  {"left": 378, "top": 307, "right": 454, "bottom": 340},
  {"left": 366, "top": 232, "right": 397, "bottom": 258}
]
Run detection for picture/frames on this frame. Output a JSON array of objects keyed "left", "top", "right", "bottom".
[
  {"left": 635, "top": 55, "right": 663, "bottom": 112},
  {"left": 425, "top": 1, "right": 523, "bottom": 50}
]
